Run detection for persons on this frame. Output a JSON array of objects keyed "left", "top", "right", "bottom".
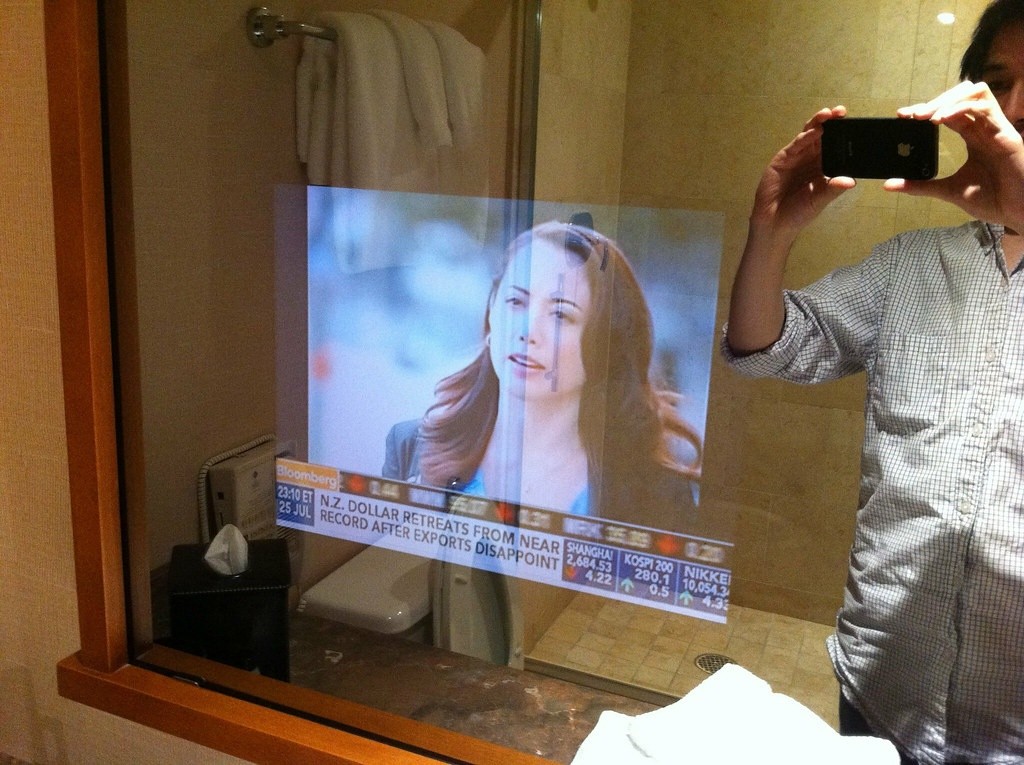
[
  {"left": 382, "top": 219, "right": 703, "bottom": 532},
  {"left": 720, "top": 2, "right": 1022, "bottom": 765}
]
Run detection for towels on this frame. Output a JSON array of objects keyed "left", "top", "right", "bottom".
[
  {"left": 569, "top": 658, "right": 901, "bottom": 765},
  {"left": 293, "top": 9, "right": 493, "bottom": 276}
]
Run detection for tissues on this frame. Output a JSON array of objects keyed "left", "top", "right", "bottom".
[{"left": 168, "top": 538, "right": 298, "bottom": 688}]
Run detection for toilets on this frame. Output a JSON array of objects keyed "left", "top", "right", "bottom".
[{"left": 302, "top": 510, "right": 525, "bottom": 674}]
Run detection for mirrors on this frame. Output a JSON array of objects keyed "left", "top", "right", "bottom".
[{"left": 42, "top": 0, "right": 1024, "bottom": 765}]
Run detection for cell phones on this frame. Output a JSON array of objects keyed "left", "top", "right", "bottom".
[{"left": 820, "top": 117, "right": 941, "bottom": 181}]
